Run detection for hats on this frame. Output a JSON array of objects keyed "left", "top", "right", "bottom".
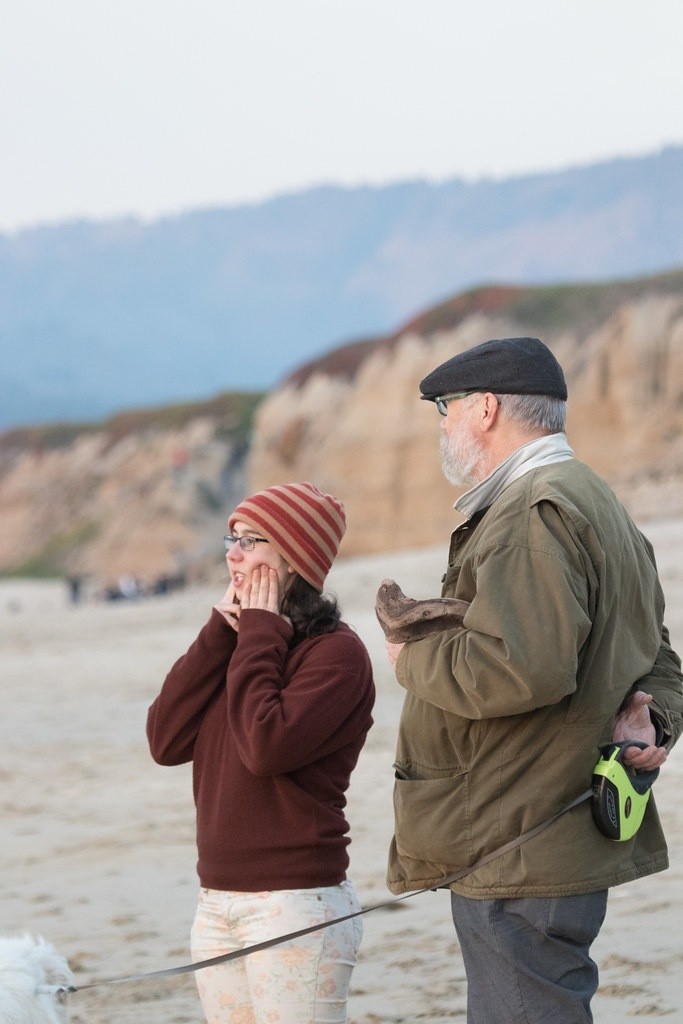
[
  {"left": 419, "top": 337, "right": 568, "bottom": 402},
  {"left": 227, "top": 481, "right": 347, "bottom": 593}
]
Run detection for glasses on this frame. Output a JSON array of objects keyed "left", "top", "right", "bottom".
[
  {"left": 223, "top": 534, "right": 269, "bottom": 551},
  {"left": 435, "top": 388, "right": 477, "bottom": 416}
]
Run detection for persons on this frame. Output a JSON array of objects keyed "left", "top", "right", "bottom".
[
  {"left": 386, "top": 338, "right": 683, "bottom": 1024},
  {"left": 145, "top": 482, "right": 376, "bottom": 1024}
]
